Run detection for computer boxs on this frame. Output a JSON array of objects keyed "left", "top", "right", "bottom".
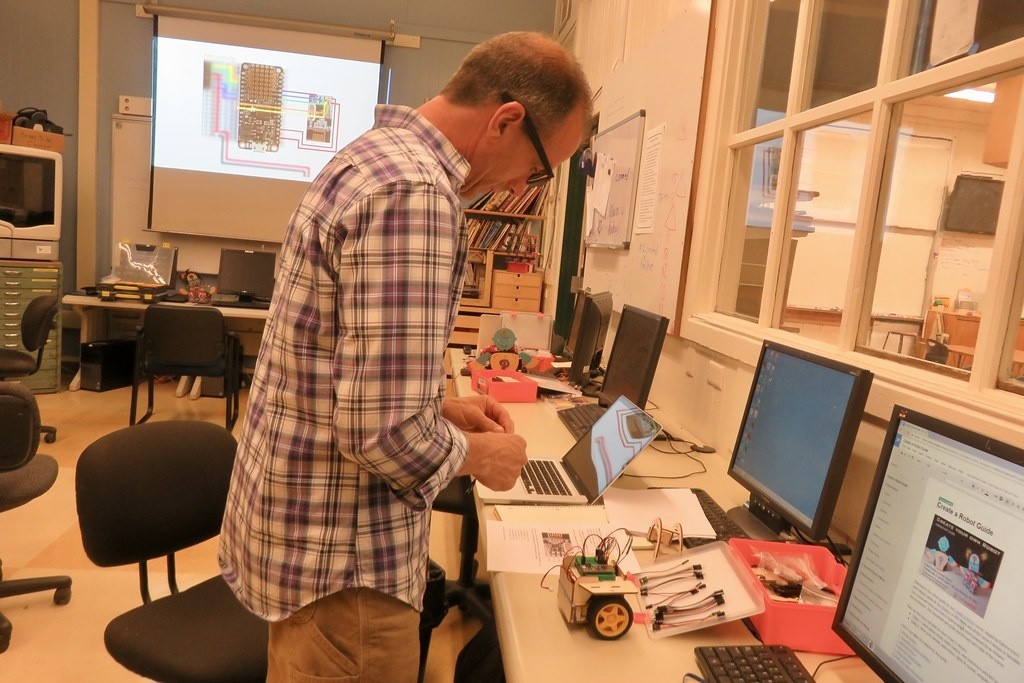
[
  {"left": 79, "top": 339, "right": 135, "bottom": 392},
  {"left": 201, "top": 335, "right": 241, "bottom": 398}
]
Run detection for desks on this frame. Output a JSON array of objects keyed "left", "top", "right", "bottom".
[
  {"left": 449, "top": 346, "right": 913, "bottom": 683},
  {"left": 1011, "top": 349, "right": 1024, "bottom": 378},
  {"left": 921, "top": 309, "right": 1024, "bottom": 366},
  {"left": 62, "top": 289, "right": 272, "bottom": 401},
  {"left": 945, "top": 344, "right": 975, "bottom": 369}
]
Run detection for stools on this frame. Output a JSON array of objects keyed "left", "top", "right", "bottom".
[{"left": 881, "top": 330, "right": 918, "bottom": 355}]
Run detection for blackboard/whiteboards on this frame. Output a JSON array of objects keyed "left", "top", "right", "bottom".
[
  {"left": 577, "top": 0, "right": 718, "bottom": 336},
  {"left": 582, "top": 108, "right": 647, "bottom": 250}
]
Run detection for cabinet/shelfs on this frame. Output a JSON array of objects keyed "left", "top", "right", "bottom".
[
  {"left": 447, "top": 165, "right": 558, "bottom": 348},
  {"left": 0, "top": 260, "right": 65, "bottom": 396}
]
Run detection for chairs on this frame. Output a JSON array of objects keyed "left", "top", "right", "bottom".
[
  {"left": 129, "top": 303, "right": 244, "bottom": 434},
  {"left": 430, "top": 476, "right": 494, "bottom": 639},
  {"left": 0, "top": 382, "right": 72, "bottom": 654},
  {"left": 0, "top": 296, "right": 57, "bottom": 444},
  {"left": 76, "top": 418, "right": 269, "bottom": 682}
]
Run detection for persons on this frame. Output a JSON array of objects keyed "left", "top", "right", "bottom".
[{"left": 216, "top": 31, "right": 593, "bottom": 683}]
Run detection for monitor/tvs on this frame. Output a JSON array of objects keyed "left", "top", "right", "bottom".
[
  {"left": 724, "top": 339, "right": 874, "bottom": 544},
  {"left": 0, "top": 144, "right": 63, "bottom": 261},
  {"left": 830, "top": 402, "right": 1024, "bottom": 683},
  {"left": 944, "top": 175, "right": 1004, "bottom": 235},
  {"left": 217, "top": 248, "right": 276, "bottom": 302},
  {"left": 563, "top": 288, "right": 613, "bottom": 398},
  {"left": 597, "top": 304, "right": 671, "bottom": 412}
]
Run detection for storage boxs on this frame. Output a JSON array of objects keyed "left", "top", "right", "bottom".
[
  {"left": 0, "top": 112, "right": 16, "bottom": 144},
  {"left": 11, "top": 126, "right": 65, "bottom": 153}
]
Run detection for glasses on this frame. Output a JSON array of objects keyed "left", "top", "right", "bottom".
[{"left": 500, "top": 90, "right": 554, "bottom": 187}]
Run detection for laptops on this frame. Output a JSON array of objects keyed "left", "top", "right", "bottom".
[{"left": 475, "top": 396, "right": 663, "bottom": 504}]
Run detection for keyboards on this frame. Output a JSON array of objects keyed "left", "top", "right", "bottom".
[
  {"left": 557, "top": 404, "right": 607, "bottom": 442},
  {"left": 211, "top": 300, "right": 269, "bottom": 309},
  {"left": 646, "top": 487, "right": 752, "bottom": 548},
  {"left": 693, "top": 645, "right": 818, "bottom": 683}
]
